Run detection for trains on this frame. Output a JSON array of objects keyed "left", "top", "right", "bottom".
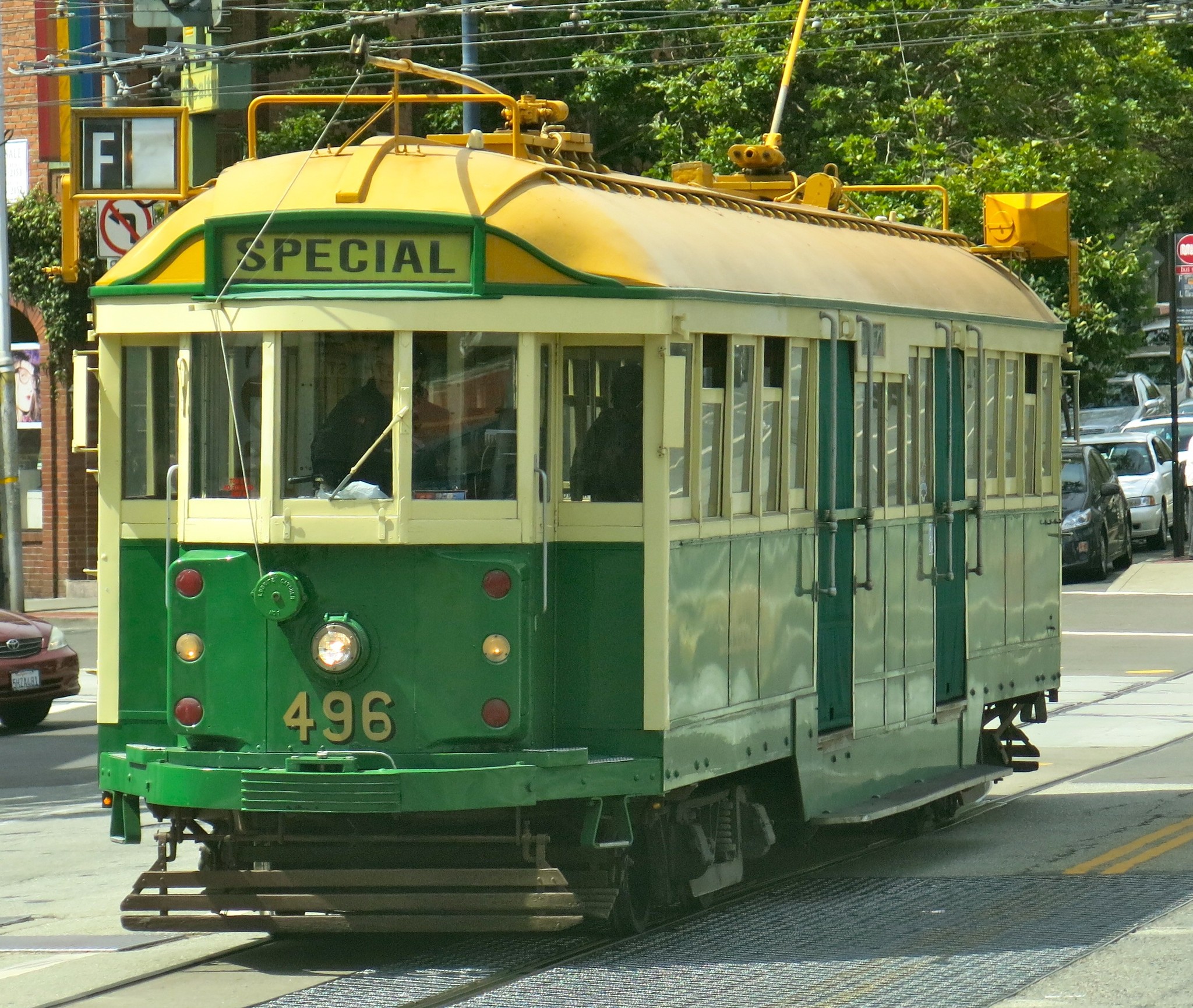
[{"left": 86, "top": 101, "right": 1076, "bottom": 938}]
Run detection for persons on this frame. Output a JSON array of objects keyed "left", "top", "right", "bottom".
[
  {"left": 311, "top": 339, "right": 451, "bottom": 500},
  {"left": 570, "top": 364, "right": 644, "bottom": 504}
]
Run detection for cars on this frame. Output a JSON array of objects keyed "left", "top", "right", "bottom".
[
  {"left": 1061, "top": 433, "right": 1189, "bottom": 551},
  {"left": 1059, "top": 444, "right": 1132, "bottom": 584},
  {"left": 1123, "top": 347, "right": 1193, "bottom": 413},
  {"left": 1122, "top": 413, "right": 1193, "bottom": 486},
  {"left": 1062, "top": 372, "right": 1167, "bottom": 434},
  {"left": 0, "top": 609, "right": 80, "bottom": 734}
]
